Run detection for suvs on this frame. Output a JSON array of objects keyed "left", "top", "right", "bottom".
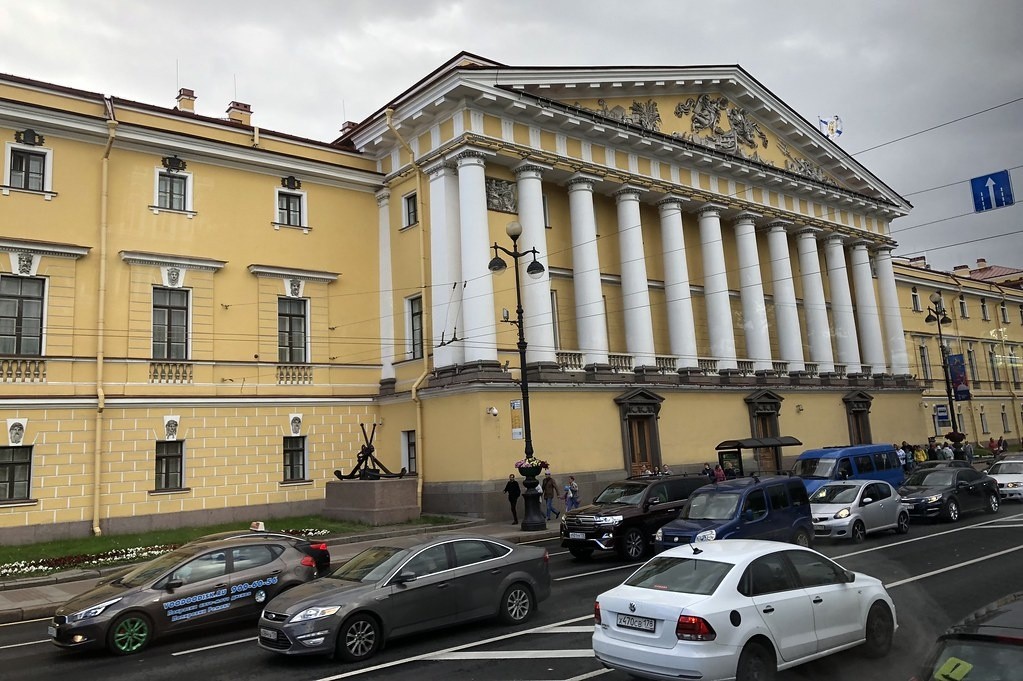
[
  {"left": 558, "top": 471, "right": 712, "bottom": 562},
  {"left": 654, "top": 472, "right": 817, "bottom": 557}
]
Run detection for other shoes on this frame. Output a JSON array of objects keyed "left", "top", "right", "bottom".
[
  {"left": 512, "top": 521, "right": 518, "bottom": 525},
  {"left": 547, "top": 518, "right": 551, "bottom": 521},
  {"left": 556, "top": 512, "right": 560, "bottom": 519}
]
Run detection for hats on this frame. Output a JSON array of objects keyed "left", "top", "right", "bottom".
[{"left": 937, "top": 446, "right": 941, "bottom": 449}]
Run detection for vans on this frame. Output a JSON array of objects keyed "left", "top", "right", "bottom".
[{"left": 790, "top": 442, "right": 908, "bottom": 497}]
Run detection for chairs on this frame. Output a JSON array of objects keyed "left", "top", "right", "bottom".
[{"left": 755, "top": 564, "right": 773, "bottom": 594}]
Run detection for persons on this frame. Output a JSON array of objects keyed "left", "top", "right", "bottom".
[
  {"left": 702, "top": 463, "right": 715, "bottom": 477},
  {"left": 749, "top": 472, "right": 757, "bottom": 477},
  {"left": 542, "top": 471, "right": 561, "bottom": 521},
  {"left": 997, "top": 436, "right": 1008, "bottom": 453},
  {"left": 988, "top": 437, "right": 998, "bottom": 458},
  {"left": 535, "top": 480, "right": 546, "bottom": 518},
  {"left": 652, "top": 466, "right": 662, "bottom": 476},
  {"left": 1021, "top": 436, "right": 1023, "bottom": 453},
  {"left": 893, "top": 441, "right": 973, "bottom": 476},
  {"left": 503, "top": 474, "right": 520, "bottom": 525},
  {"left": 662, "top": 464, "right": 674, "bottom": 476},
  {"left": 724, "top": 462, "right": 736, "bottom": 480},
  {"left": 560, "top": 476, "right": 580, "bottom": 512},
  {"left": 640, "top": 464, "right": 652, "bottom": 475},
  {"left": 713, "top": 465, "right": 726, "bottom": 482}
]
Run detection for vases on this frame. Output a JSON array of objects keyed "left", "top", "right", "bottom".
[{"left": 518, "top": 467, "right": 542, "bottom": 475}]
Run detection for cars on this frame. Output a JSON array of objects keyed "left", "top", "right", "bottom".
[
  {"left": 893, "top": 467, "right": 1002, "bottom": 522},
  {"left": 256, "top": 530, "right": 554, "bottom": 664},
  {"left": 46, "top": 538, "right": 319, "bottom": 657},
  {"left": 95, "top": 521, "right": 331, "bottom": 589},
  {"left": 980, "top": 451, "right": 1023, "bottom": 505},
  {"left": 904, "top": 591, "right": 1023, "bottom": 681},
  {"left": 897, "top": 459, "right": 977, "bottom": 489},
  {"left": 591, "top": 537, "right": 900, "bottom": 681},
  {"left": 808, "top": 480, "right": 910, "bottom": 541}
]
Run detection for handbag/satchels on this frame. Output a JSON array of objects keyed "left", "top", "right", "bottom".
[
  {"left": 993, "top": 447, "right": 998, "bottom": 451},
  {"left": 576, "top": 499, "right": 581, "bottom": 504},
  {"left": 999, "top": 446, "right": 1004, "bottom": 452}
]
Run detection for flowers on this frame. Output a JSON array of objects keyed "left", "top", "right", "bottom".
[
  {"left": 515, "top": 457, "right": 549, "bottom": 468},
  {"left": 945, "top": 432, "right": 965, "bottom": 441}
]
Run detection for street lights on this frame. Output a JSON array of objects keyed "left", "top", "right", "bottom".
[
  {"left": 487, "top": 222, "right": 548, "bottom": 535},
  {"left": 925, "top": 291, "right": 965, "bottom": 461}
]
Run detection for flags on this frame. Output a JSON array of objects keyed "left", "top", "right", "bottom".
[{"left": 820, "top": 115, "right": 843, "bottom": 140}]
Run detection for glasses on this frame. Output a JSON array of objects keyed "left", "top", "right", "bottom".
[{"left": 545, "top": 474, "right": 548, "bottom": 475}]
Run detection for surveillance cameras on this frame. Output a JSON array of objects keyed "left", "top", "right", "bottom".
[{"left": 492, "top": 408, "right": 498, "bottom": 416}]
